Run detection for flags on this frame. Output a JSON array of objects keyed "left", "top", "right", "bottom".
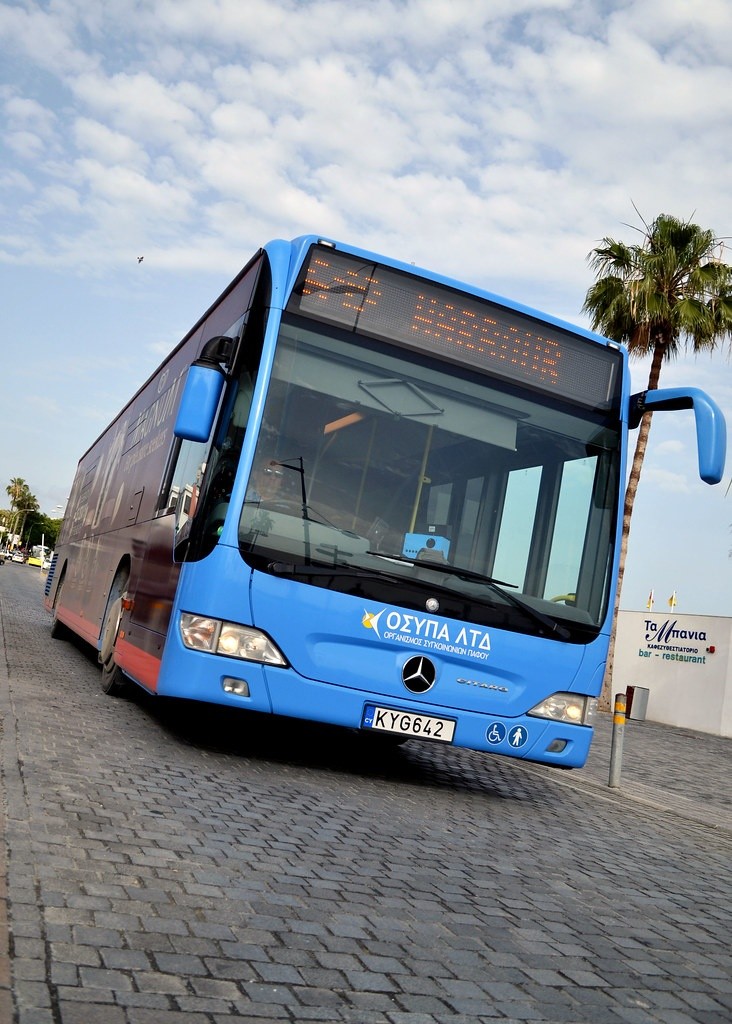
[
  {"left": 668, "top": 595, "right": 676, "bottom": 606},
  {"left": 646, "top": 591, "right": 655, "bottom": 608}
]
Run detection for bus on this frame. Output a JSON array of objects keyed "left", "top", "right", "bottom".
[{"left": 42, "top": 233, "right": 728, "bottom": 772}]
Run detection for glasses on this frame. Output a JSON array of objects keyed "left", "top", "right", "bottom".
[{"left": 261, "top": 467, "right": 285, "bottom": 479}]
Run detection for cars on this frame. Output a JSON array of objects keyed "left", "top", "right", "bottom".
[{"left": 0, "top": 545, "right": 55, "bottom": 570}]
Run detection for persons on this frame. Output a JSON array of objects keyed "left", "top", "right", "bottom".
[{"left": 225, "top": 451, "right": 293, "bottom": 511}]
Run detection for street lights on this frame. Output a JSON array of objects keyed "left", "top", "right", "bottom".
[
  {"left": 25, "top": 523, "right": 45, "bottom": 550},
  {"left": 5, "top": 509, "right": 35, "bottom": 549}
]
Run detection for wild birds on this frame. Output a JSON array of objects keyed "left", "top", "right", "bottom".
[{"left": 136, "top": 256, "right": 145, "bottom": 266}]
